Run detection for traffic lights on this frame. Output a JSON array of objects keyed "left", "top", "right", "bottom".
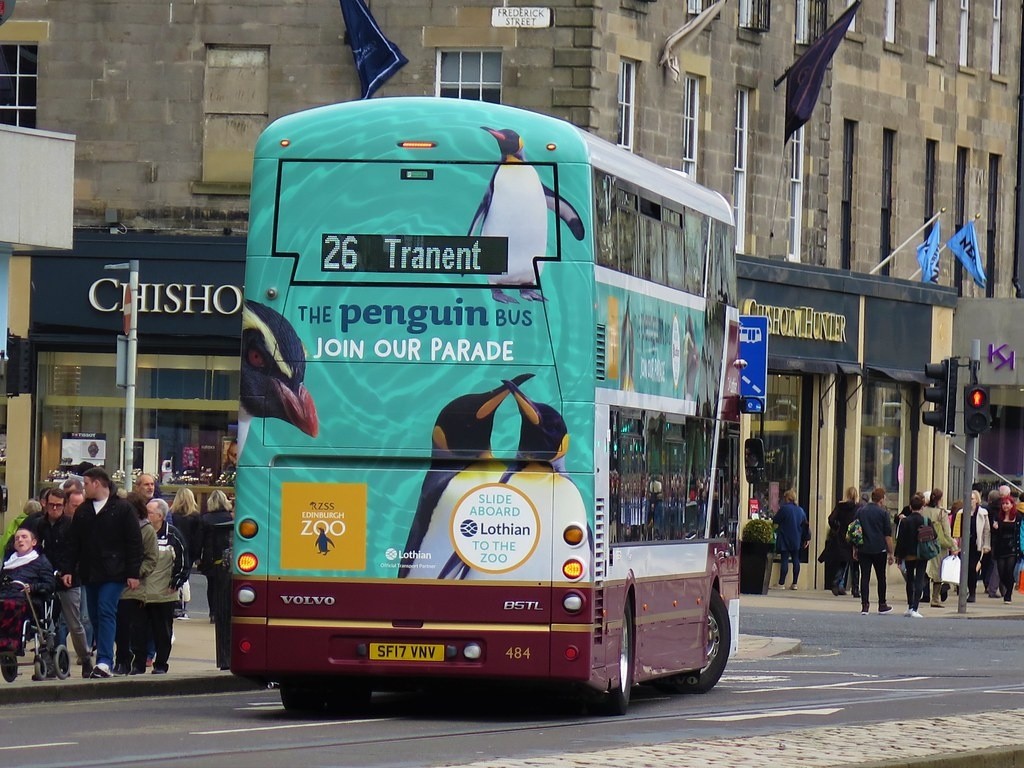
[
  {"left": 923, "top": 357, "right": 958, "bottom": 432},
  {"left": 964, "top": 385, "right": 991, "bottom": 434}
]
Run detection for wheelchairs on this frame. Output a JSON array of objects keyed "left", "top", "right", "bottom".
[{"left": 0, "top": 570, "right": 71, "bottom": 682}]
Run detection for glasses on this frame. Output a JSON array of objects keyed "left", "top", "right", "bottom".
[{"left": 47, "top": 502, "right": 64, "bottom": 512}]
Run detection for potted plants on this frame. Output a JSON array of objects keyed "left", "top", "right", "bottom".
[{"left": 741, "top": 520, "right": 777, "bottom": 596}]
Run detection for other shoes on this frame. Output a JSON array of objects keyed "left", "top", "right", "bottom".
[
  {"left": 790, "top": 584, "right": 798, "bottom": 590},
  {"left": 967, "top": 594, "right": 976, "bottom": 603},
  {"left": 989, "top": 594, "right": 1002, "bottom": 598},
  {"left": 177, "top": 612, "right": 191, "bottom": 620},
  {"left": 941, "top": 583, "right": 950, "bottom": 602},
  {"left": 920, "top": 595, "right": 930, "bottom": 604},
  {"left": 1004, "top": 594, "right": 1012, "bottom": 604},
  {"left": 32, "top": 650, "right": 169, "bottom": 681},
  {"left": 769, "top": 583, "right": 785, "bottom": 590}
]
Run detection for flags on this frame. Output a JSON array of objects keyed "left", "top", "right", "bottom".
[
  {"left": 340, "top": 0, "right": 409, "bottom": 99},
  {"left": 663, "top": 0, "right": 726, "bottom": 84},
  {"left": 784, "top": 2, "right": 860, "bottom": 146},
  {"left": 946, "top": 221, "right": 987, "bottom": 288},
  {"left": 917, "top": 218, "right": 940, "bottom": 283}
]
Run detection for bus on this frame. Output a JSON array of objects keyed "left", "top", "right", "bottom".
[{"left": 232, "top": 97, "right": 766, "bottom": 716}]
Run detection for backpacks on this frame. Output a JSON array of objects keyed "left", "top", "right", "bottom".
[
  {"left": 846, "top": 518, "right": 863, "bottom": 545},
  {"left": 917, "top": 517, "right": 938, "bottom": 559}
]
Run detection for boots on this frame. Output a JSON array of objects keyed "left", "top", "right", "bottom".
[{"left": 931, "top": 584, "right": 945, "bottom": 608}]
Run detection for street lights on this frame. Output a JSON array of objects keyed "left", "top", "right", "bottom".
[{"left": 104, "top": 260, "right": 139, "bottom": 493}]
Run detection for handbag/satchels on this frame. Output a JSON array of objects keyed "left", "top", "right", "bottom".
[
  {"left": 1013, "top": 557, "right": 1024, "bottom": 595},
  {"left": 942, "top": 554, "right": 961, "bottom": 584}
]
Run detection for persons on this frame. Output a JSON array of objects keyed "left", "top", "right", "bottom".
[
  {"left": 611, "top": 469, "right": 717, "bottom": 541},
  {"left": 772, "top": 489, "right": 810, "bottom": 590},
  {"left": 828, "top": 485, "right": 1024, "bottom": 618},
  {"left": 0, "top": 467, "right": 234, "bottom": 680},
  {"left": 223, "top": 440, "right": 237, "bottom": 471}
]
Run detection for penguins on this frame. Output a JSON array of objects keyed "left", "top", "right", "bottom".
[
  {"left": 236, "top": 298, "right": 319, "bottom": 459},
  {"left": 399, "top": 374, "right": 596, "bottom": 583},
  {"left": 315, "top": 528, "right": 335, "bottom": 555},
  {"left": 467, "top": 126, "right": 586, "bottom": 302}
]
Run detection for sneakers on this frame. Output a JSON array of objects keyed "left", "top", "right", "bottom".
[
  {"left": 912, "top": 611, "right": 923, "bottom": 618},
  {"left": 879, "top": 602, "right": 893, "bottom": 614},
  {"left": 861, "top": 603, "right": 870, "bottom": 615},
  {"left": 903, "top": 609, "right": 913, "bottom": 616}
]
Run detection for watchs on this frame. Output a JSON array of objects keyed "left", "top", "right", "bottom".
[{"left": 88, "top": 441, "right": 98, "bottom": 457}]
